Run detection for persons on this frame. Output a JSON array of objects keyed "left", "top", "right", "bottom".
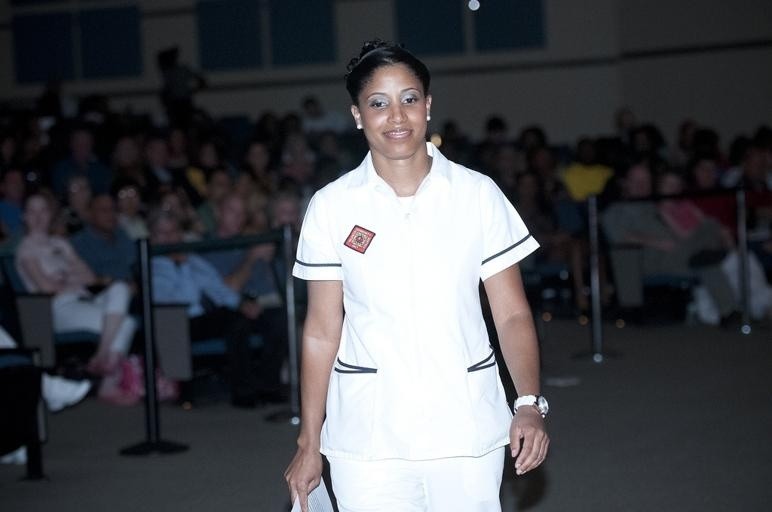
[
  {"left": 1, "top": 42, "right": 771, "bottom": 412},
  {"left": 286, "top": 40, "right": 549, "bottom": 512}
]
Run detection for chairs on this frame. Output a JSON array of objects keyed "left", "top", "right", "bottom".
[{"left": 0, "top": 200, "right": 771, "bottom": 445}]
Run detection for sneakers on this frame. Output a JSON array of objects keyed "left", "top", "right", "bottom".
[
  {"left": 0, "top": 444, "right": 31, "bottom": 466},
  {"left": 37, "top": 372, "right": 90, "bottom": 413}
]
